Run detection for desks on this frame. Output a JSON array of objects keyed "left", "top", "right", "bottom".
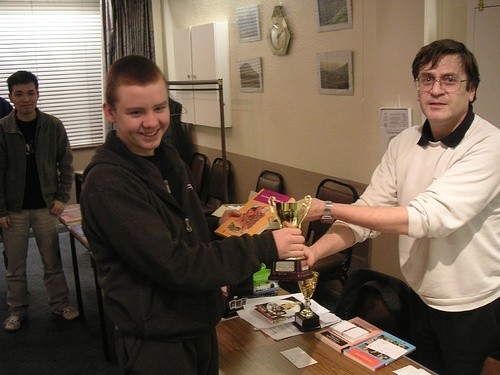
[{"left": 56, "top": 203, "right": 438, "bottom": 375}]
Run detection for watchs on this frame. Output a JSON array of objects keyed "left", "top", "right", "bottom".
[{"left": 320, "top": 201, "right": 334, "bottom": 223}]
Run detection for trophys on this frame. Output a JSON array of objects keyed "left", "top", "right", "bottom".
[
  {"left": 294, "top": 272, "right": 321, "bottom": 332},
  {"left": 268, "top": 195, "right": 314, "bottom": 281}
]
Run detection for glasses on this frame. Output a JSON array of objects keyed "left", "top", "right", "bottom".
[{"left": 414, "top": 76, "right": 469, "bottom": 92}]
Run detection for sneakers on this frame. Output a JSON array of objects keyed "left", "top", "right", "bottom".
[
  {"left": 52, "top": 303, "right": 79, "bottom": 319},
  {"left": 4, "top": 315, "right": 23, "bottom": 330}
]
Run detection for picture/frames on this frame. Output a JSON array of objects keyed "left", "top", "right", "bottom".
[
  {"left": 316, "top": 50, "right": 354, "bottom": 96},
  {"left": 235, "top": 3, "right": 261, "bottom": 42},
  {"left": 314, "top": 0, "right": 353, "bottom": 32},
  {"left": 237, "top": 57, "right": 264, "bottom": 93}
]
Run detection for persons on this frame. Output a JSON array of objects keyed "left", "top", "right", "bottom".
[
  {"left": 299, "top": 39, "right": 500, "bottom": 375},
  {"left": 79, "top": 56, "right": 305, "bottom": 375},
  {"left": 0, "top": 71, "right": 79, "bottom": 330}
]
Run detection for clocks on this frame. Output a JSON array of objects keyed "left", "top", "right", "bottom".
[{"left": 265, "top": 5, "right": 291, "bottom": 56}]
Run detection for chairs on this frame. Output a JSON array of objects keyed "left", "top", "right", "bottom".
[{"left": 189, "top": 153, "right": 417, "bottom": 358}]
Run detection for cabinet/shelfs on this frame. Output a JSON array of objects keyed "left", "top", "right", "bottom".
[{"left": 172, "top": 22, "right": 231, "bottom": 127}]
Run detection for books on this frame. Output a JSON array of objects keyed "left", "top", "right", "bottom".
[
  {"left": 214, "top": 189, "right": 295, "bottom": 239},
  {"left": 315, "top": 317, "right": 416, "bottom": 371},
  {"left": 254, "top": 296, "right": 305, "bottom": 324}
]
